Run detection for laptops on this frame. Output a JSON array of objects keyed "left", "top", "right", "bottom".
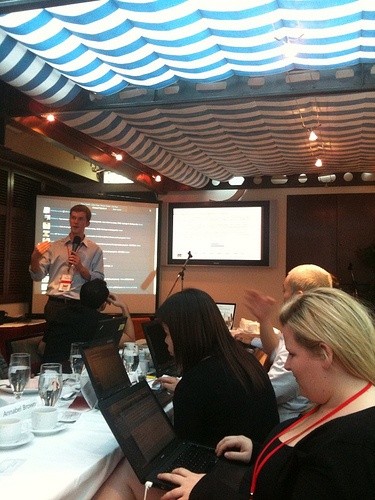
[{"left": 79, "top": 303, "right": 237, "bottom": 491}]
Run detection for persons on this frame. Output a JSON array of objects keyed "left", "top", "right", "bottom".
[
  {"left": 235, "top": 333, "right": 273, "bottom": 373},
  {"left": 28, "top": 205, "right": 104, "bottom": 364},
  {"left": 157, "top": 287, "right": 375, "bottom": 500},
  {"left": 46, "top": 279, "right": 135, "bottom": 374},
  {"left": 243, "top": 263, "right": 333, "bottom": 420},
  {"left": 153, "top": 288, "right": 280, "bottom": 451}
]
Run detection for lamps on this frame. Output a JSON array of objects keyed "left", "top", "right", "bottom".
[
  {"left": 151, "top": 169, "right": 162, "bottom": 183},
  {"left": 212, "top": 173, "right": 375, "bottom": 187},
  {"left": 295, "top": 96, "right": 325, "bottom": 168},
  {"left": 111, "top": 150, "right": 124, "bottom": 161}
]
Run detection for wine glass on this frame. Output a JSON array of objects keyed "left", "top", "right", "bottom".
[{"left": 8, "top": 353, "right": 31, "bottom": 402}]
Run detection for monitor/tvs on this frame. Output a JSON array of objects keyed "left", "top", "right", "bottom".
[{"left": 167, "top": 200, "right": 270, "bottom": 267}]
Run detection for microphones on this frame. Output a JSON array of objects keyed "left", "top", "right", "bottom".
[{"left": 67, "top": 235, "right": 82, "bottom": 273}]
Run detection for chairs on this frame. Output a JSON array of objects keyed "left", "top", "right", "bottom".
[
  {"left": 6, "top": 332, "right": 43, "bottom": 372},
  {"left": 132, "top": 318, "right": 151, "bottom": 340}
]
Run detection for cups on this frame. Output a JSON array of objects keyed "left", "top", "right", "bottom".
[
  {"left": 31, "top": 406, "right": 58, "bottom": 430},
  {"left": 38, "top": 363, "right": 63, "bottom": 407},
  {"left": 140, "top": 360, "right": 150, "bottom": 372},
  {"left": 70, "top": 343, "right": 85, "bottom": 392},
  {"left": 123, "top": 342, "right": 139, "bottom": 372},
  {"left": 0, "top": 418, "right": 21, "bottom": 443}
]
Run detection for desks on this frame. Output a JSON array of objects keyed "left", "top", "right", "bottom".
[
  {"left": 0, "top": 320, "right": 48, "bottom": 369},
  {"left": 0, "top": 365, "right": 173, "bottom": 500}
]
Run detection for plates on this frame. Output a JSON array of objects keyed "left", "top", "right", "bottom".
[
  {"left": 27, "top": 423, "right": 67, "bottom": 436},
  {"left": 0, "top": 385, "right": 38, "bottom": 394},
  {"left": 0, "top": 432, "right": 34, "bottom": 449}
]
